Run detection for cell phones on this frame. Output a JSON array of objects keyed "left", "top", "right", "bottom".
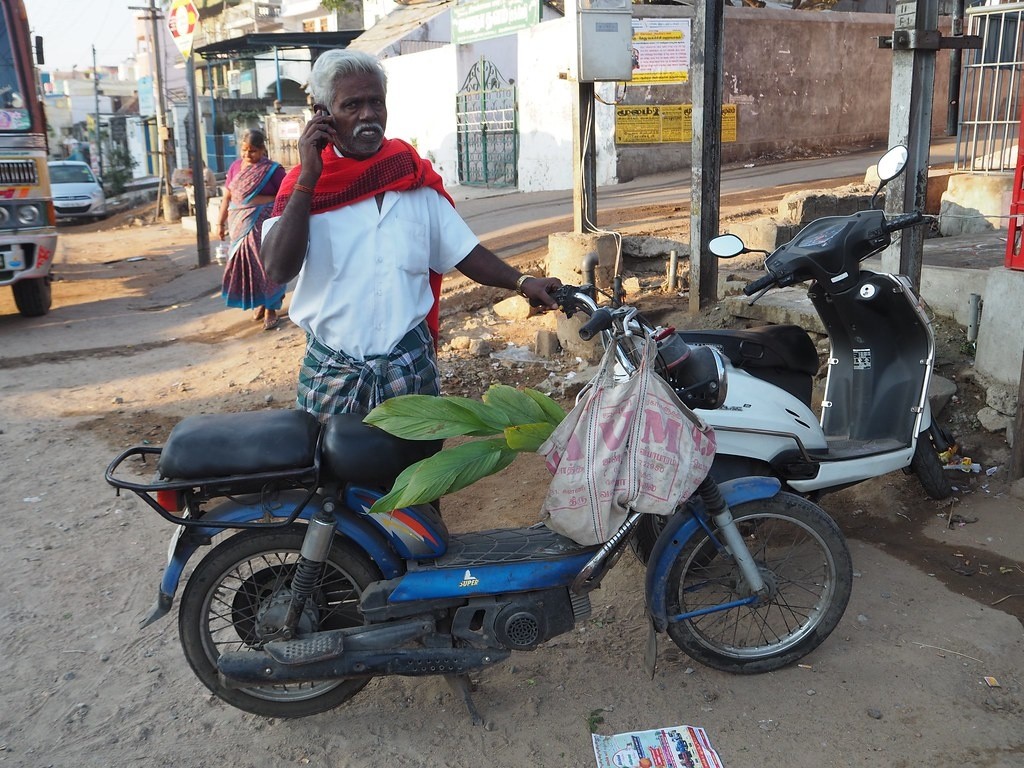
[{"left": 317, "top": 110, "right": 329, "bottom": 148}]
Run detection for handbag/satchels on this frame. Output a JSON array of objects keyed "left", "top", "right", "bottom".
[{"left": 536, "top": 325, "right": 717, "bottom": 546}]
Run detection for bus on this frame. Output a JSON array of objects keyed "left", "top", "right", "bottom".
[{"left": 0, "top": 2, "right": 59, "bottom": 316}]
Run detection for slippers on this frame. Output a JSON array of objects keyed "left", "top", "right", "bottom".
[
  {"left": 262, "top": 318, "right": 279, "bottom": 330},
  {"left": 253, "top": 311, "right": 265, "bottom": 320}
]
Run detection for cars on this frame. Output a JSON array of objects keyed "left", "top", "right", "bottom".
[{"left": 48, "top": 160, "right": 108, "bottom": 222}]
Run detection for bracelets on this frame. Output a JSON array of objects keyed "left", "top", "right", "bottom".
[
  {"left": 516, "top": 274, "right": 536, "bottom": 298},
  {"left": 215, "top": 223, "right": 225, "bottom": 226},
  {"left": 293, "top": 183, "right": 315, "bottom": 194}
]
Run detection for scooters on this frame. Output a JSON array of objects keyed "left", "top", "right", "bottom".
[{"left": 571, "top": 138, "right": 951, "bottom": 572}]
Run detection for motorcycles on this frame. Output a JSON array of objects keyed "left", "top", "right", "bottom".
[{"left": 105, "top": 286, "right": 853, "bottom": 726}]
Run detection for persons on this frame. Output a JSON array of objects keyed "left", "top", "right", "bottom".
[
  {"left": 258, "top": 47, "right": 579, "bottom": 520},
  {"left": 202, "top": 159, "right": 217, "bottom": 207},
  {"left": 216, "top": 129, "right": 287, "bottom": 330}
]
